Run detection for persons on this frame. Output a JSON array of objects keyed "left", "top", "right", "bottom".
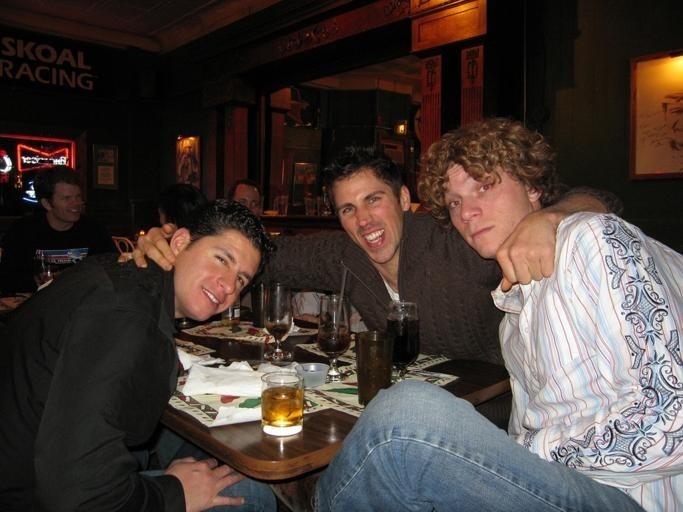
[
  {"left": 228, "top": 179, "right": 261, "bottom": 220},
  {"left": 157, "top": 184, "right": 209, "bottom": 229},
  {"left": 116, "top": 145, "right": 627, "bottom": 433},
  {"left": 0, "top": 166, "right": 110, "bottom": 292},
  {"left": 310, "top": 113, "right": 683, "bottom": 512},
  {"left": 0, "top": 199, "right": 278, "bottom": 512}
]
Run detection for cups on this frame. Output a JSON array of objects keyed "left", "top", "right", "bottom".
[
  {"left": 221, "top": 293, "right": 241, "bottom": 327},
  {"left": 354, "top": 331, "right": 394, "bottom": 407},
  {"left": 275, "top": 193, "right": 289, "bottom": 216},
  {"left": 303, "top": 187, "right": 332, "bottom": 214},
  {"left": 43, "top": 264, "right": 58, "bottom": 286},
  {"left": 261, "top": 370, "right": 303, "bottom": 436}
]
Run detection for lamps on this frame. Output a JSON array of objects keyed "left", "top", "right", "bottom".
[{"left": 396, "top": 118, "right": 408, "bottom": 139}]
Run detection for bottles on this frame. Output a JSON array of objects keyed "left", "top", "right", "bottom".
[{"left": 251, "top": 266, "right": 269, "bottom": 328}]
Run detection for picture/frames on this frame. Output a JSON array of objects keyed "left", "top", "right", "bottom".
[{"left": 627, "top": 50, "right": 683, "bottom": 184}]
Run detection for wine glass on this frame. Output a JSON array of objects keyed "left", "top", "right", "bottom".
[
  {"left": 384, "top": 300, "right": 420, "bottom": 384},
  {"left": 262, "top": 283, "right": 292, "bottom": 361},
  {"left": 318, "top": 294, "right": 349, "bottom": 380}
]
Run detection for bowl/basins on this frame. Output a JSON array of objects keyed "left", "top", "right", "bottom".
[{"left": 294, "top": 362, "right": 330, "bottom": 386}]
